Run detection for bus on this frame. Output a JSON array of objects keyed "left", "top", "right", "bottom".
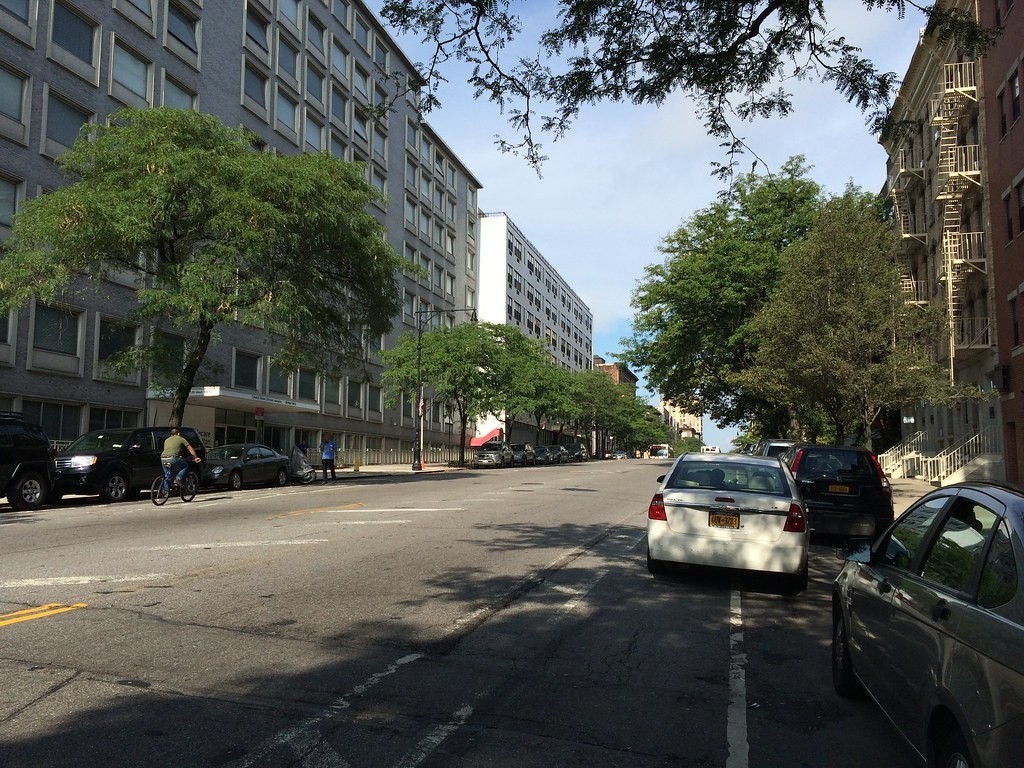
[
  {"left": 701, "top": 446, "right": 721, "bottom": 453},
  {"left": 649, "top": 443, "right": 674, "bottom": 459}
]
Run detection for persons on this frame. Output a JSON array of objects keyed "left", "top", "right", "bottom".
[
  {"left": 299, "top": 440, "right": 309, "bottom": 454},
  {"left": 320, "top": 435, "right": 338, "bottom": 484},
  {"left": 161, "top": 430, "right": 201, "bottom": 496}
]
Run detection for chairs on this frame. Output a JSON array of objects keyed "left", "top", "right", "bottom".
[
  {"left": 687, "top": 472, "right": 712, "bottom": 487},
  {"left": 748, "top": 476, "right": 776, "bottom": 491}
]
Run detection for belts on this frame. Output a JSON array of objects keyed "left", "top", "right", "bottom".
[{"left": 161, "top": 456, "right": 174, "bottom": 458}]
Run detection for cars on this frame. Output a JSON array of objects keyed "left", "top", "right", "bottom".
[
  {"left": 610, "top": 451, "right": 628, "bottom": 460},
  {"left": 645, "top": 452, "right": 809, "bottom": 596},
  {"left": 830, "top": 480, "right": 1024, "bottom": 768},
  {"left": 204, "top": 444, "right": 290, "bottom": 491},
  {"left": 533, "top": 443, "right": 589, "bottom": 465}
]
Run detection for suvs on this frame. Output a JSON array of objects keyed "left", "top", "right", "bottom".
[
  {"left": 55, "top": 426, "right": 207, "bottom": 503},
  {"left": 0, "top": 410, "right": 57, "bottom": 511},
  {"left": 740, "top": 439, "right": 895, "bottom": 537},
  {"left": 473, "top": 440, "right": 514, "bottom": 468},
  {"left": 510, "top": 442, "right": 536, "bottom": 466}
]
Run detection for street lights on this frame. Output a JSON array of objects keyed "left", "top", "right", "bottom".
[{"left": 412, "top": 300, "right": 478, "bottom": 471}]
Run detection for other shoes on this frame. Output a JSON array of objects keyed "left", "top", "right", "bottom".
[
  {"left": 176, "top": 480, "right": 187, "bottom": 491},
  {"left": 330, "top": 481, "right": 337, "bottom": 485},
  {"left": 322, "top": 482, "right": 328, "bottom": 485}
]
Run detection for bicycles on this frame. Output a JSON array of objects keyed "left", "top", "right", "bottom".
[{"left": 150, "top": 456, "right": 198, "bottom": 506}]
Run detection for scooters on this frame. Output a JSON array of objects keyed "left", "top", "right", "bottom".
[{"left": 274, "top": 445, "right": 317, "bottom": 485}]
